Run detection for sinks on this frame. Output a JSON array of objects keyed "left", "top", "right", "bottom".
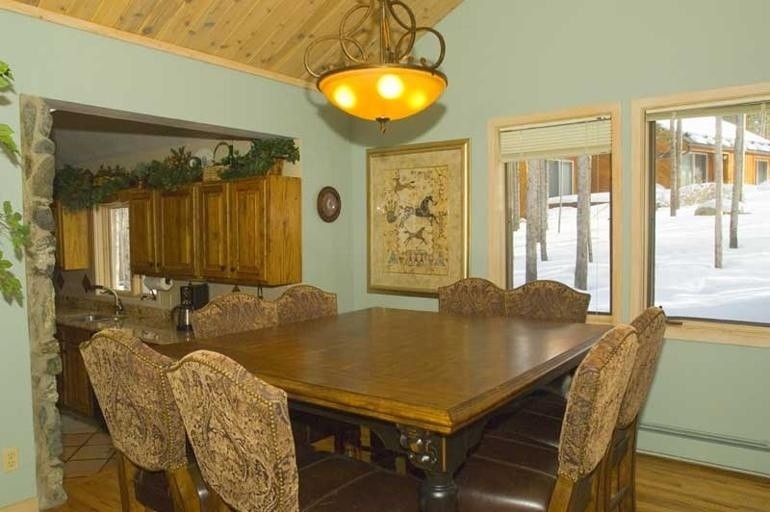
[{"left": 68, "top": 315, "right": 113, "bottom": 323}]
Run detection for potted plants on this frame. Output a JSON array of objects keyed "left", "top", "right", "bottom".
[{"left": 248, "top": 137, "right": 301, "bottom": 176}]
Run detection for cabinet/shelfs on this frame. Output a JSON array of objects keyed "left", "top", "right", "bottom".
[
  {"left": 50, "top": 200, "right": 93, "bottom": 270},
  {"left": 198, "top": 178, "right": 302, "bottom": 287},
  {"left": 129, "top": 184, "right": 199, "bottom": 280}
]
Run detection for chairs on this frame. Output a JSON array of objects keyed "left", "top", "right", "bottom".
[
  {"left": 79, "top": 330, "right": 218, "bottom": 512},
  {"left": 439, "top": 277, "right": 505, "bottom": 316},
  {"left": 505, "top": 280, "right": 591, "bottom": 397},
  {"left": 274, "top": 284, "right": 356, "bottom": 455},
  {"left": 165, "top": 351, "right": 421, "bottom": 512},
  {"left": 456, "top": 325, "right": 638, "bottom": 512},
  {"left": 189, "top": 292, "right": 279, "bottom": 337},
  {"left": 490, "top": 307, "right": 667, "bottom": 511}
]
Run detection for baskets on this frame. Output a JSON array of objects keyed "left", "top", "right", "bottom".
[
  {"left": 203, "top": 142, "right": 235, "bottom": 183},
  {"left": 264, "top": 154, "right": 286, "bottom": 176}
]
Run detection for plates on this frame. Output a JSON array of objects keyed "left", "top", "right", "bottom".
[{"left": 317, "top": 186, "right": 341, "bottom": 222}]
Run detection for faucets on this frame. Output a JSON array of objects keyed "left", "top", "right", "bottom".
[{"left": 88, "top": 285, "right": 118, "bottom": 315}]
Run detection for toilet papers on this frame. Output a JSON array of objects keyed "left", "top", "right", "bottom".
[{"left": 144, "top": 276, "right": 173, "bottom": 291}]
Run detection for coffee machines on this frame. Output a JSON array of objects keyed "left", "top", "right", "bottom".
[{"left": 171, "top": 283, "right": 209, "bottom": 331}]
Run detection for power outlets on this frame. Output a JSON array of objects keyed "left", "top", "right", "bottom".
[{"left": 1, "top": 449, "right": 19, "bottom": 473}]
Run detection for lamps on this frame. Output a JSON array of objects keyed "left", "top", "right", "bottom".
[{"left": 302, "top": 0, "right": 449, "bottom": 132}]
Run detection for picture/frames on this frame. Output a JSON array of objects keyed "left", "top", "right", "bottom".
[{"left": 366, "top": 138, "right": 472, "bottom": 300}]
